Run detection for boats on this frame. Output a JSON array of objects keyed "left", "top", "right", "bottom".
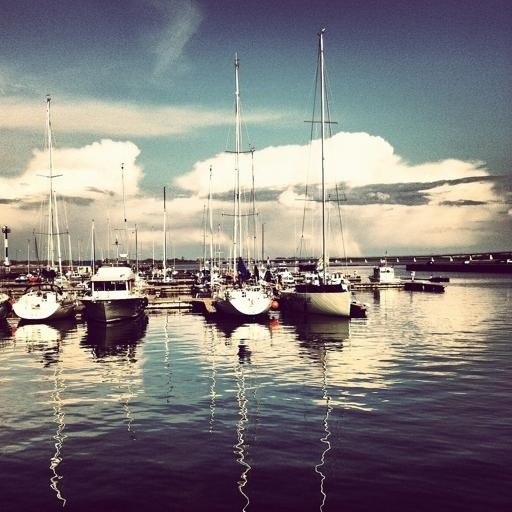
[
  {"left": 0, "top": 293, "right": 14, "bottom": 320},
  {"left": 83, "top": 313, "right": 151, "bottom": 436},
  {"left": 403, "top": 280, "right": 446, "bottom": 292},
  {"left": 83, "top": 219, "right": 150, "bottom": 326},
  {"left": 368, "top": 251, "right": 400, "bottom": 284},
  {"left": 266, "top": 260, "right": 362, "bottom": 284}
]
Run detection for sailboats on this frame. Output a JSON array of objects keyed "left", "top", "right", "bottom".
[
  {"left": 278, "top": 26, "right": 354, "bottom": 320},
  {"left": 293, "top": 315, "right": 353, "bottom": 509},
  {"left": 151, "top": 185, "right": 177, "bottom": 277},
  {"left": 215, "top": 51, "right": 273, "bottom": 322},
  {"left": 13, "top": 95, "right": 81, "bottom": 321},
  {"left": 13, "top": 319, "right": 74, "bottom": 505}
]
